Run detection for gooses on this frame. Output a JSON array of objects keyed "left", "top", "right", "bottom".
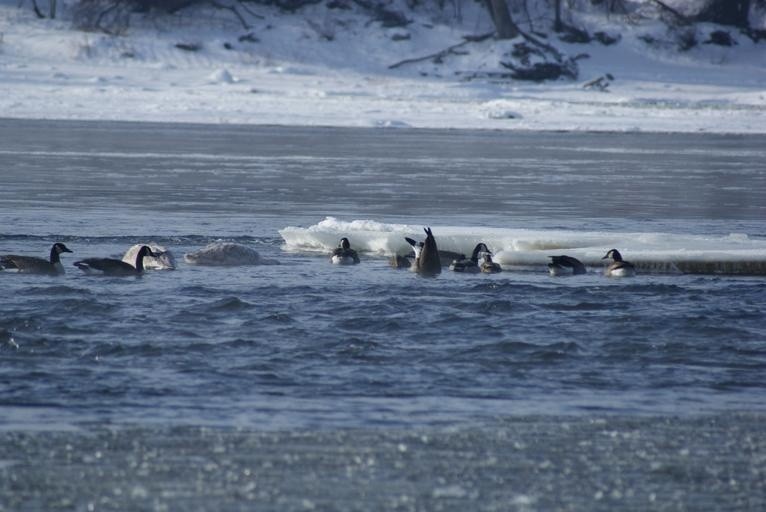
[
  {"left": 184, "top": 242, "right": 280, "bottom": 267},
  {"left": 404, "top": 227, "right": 502, "bottom": 274},
  {"left": 602, "top": 249, "right": 637, "bottom": 278},
  {"left": 74, "top": 242, "right": 175, "bottom": 278},
  {"left": 331, "top": 238, "right": 358, "bottom": 266},
  {"left": 0, "top": 241, "right": 73, "bottom": 276},
  {"left": 546, "top": 254, "right": 586, "bottom": 277}
]
